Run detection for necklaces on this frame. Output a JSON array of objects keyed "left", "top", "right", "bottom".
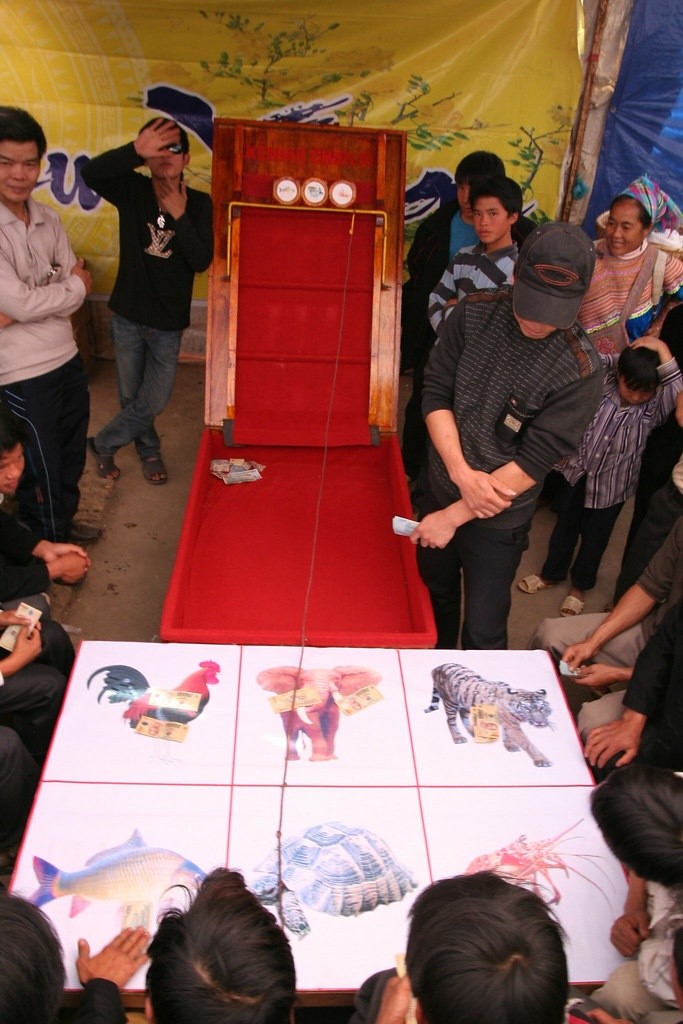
[{"left": 157, "top": 207, "right": 166, "bottom": 228}]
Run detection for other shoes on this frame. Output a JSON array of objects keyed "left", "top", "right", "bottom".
[
  {"left": 68, "top": 524, "right": 102, "bottom": 545},
  {"left": 605, "top": 598, "right": 613, "bottom": 613}
]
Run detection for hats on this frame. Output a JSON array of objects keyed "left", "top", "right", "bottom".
[{"left": 513, "top": 222, "right": 595, "bottom": 330}]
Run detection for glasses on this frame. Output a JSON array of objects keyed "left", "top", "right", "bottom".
[{"left": 166, "top": 143, "right": 184, "bottom": 154}]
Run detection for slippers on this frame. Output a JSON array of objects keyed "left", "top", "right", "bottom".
[
  {"left": 560, "top": 596, "right": 584, "bottom": 617},
  {"left": 86, "top": 435, "right": 120, "bottom": 480},
  {"left": 141, "top": 455, "right": 167, "bottom": 484},
  {"left": 517, "top": 575, "right": 559, "bottom": 593}
]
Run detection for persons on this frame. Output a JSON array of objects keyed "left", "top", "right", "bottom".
[
  {"left": 0, "top": 892, "right": 153, "bottom": 1024},
  {"left": 0, "top": 106, "right": 102, "bottom": 584},
  {"left": 0, "top": 404, "right": 91, "bottom": 624},
  {"left": 348, "top": 151, "right": 683, "bottom": 1024},
  {"left": 81, "top": 116, "right": 214, "bottom": 485},
  {"left": 145, "top": 867, "right": 296, "bottom": 1024},
  {"left": 0, "top": 608, "right": 93, "bottom": 874}
]
